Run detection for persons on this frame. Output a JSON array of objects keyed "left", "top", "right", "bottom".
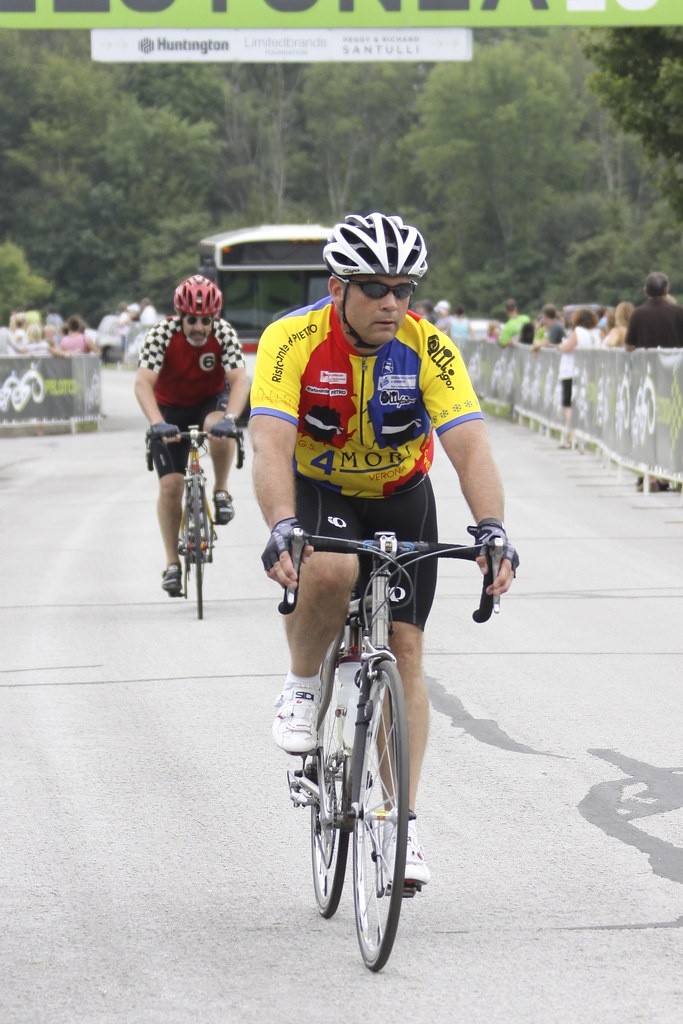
[
  {"left": 412, "top": 272, "right": 683, "bottom": 492},
  {"left": 134, "top": 275, "right": 250, "bottom": 590},
  {"left": 0, "top": 297, "right": 158, "bottom": 356},
  {"left": 248, "top": 211, "right": 520, "bottom": 889}
]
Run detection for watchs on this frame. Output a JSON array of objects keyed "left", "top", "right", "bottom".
[{"left": 224, "top": 413, "right": 238, "bottom": 422}]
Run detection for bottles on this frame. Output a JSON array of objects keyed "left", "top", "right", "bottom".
[{"left": 334, "top": 647, "right": 384, "bottom": 749}]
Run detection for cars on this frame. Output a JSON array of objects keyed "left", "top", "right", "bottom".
[{"left": 97, "top": 315, "right": 122, "bottom": 363}]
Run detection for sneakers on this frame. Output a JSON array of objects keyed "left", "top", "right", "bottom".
[
  {"left": 382, "top": 820, "right": 430, "bottom": 886},
  {"left": 273, "top": 680, "right": 321, "bottom": 755},
  {"left": 163, "top": 562, "right": 182, "bottom": 591},
  {"left": 213, "top": 489, "right": 235, "bottom": 523}
]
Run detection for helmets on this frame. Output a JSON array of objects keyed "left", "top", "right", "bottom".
[
  {"left": 323, "top": 212, "right": 429, "bottom": 278},
  {"left": 174, "top": 275, "right": 223, "bottom": 315}
]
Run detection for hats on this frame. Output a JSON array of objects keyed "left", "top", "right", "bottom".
[{"left": 433, "top": 301, "right": 449, "bottom": 311}]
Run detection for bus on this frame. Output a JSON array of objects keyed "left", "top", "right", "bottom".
[{"left": 198, "top": 224, "right": 335, "bottom": 389}]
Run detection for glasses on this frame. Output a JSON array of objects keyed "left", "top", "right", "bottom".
[
  {"left": 333, "top": 273, "right": 418, "bottom": 299},
  {"left": 182, "top": 316, "right": 212, "bottom": 326}
]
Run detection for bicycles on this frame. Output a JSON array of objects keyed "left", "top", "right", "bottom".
[
  {"left": 146, "top": 425, "right": 245, "bottom": 619},
  {"left": 278, "top": 524, "right": 495, "bottom": 971}
]
